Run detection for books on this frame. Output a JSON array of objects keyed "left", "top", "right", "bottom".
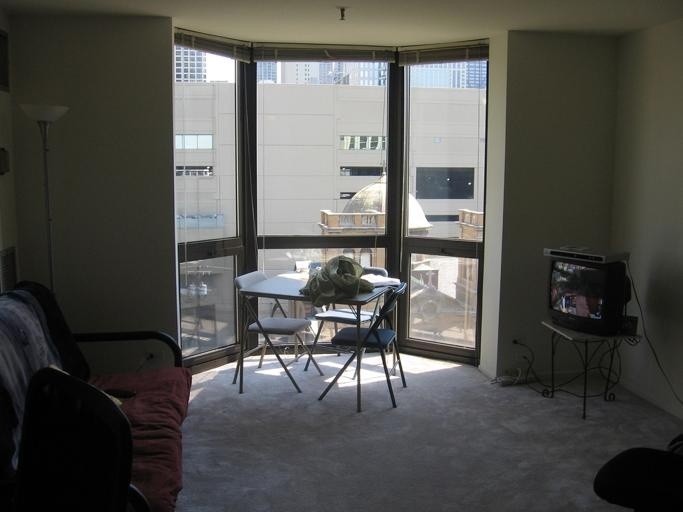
[{"left": 361, "top": 273, "right": 401, "bottom": 288}]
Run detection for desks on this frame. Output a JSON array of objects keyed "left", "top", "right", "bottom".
[{"left": 540, "top": 320, "right": 623, "bottom": 420}]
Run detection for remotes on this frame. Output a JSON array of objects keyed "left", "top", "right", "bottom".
[{"left": 104, "top": 388, "right": 137, "bottom": 399}]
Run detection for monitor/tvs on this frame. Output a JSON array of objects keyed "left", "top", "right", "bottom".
[{"left": 546, "top": 257, "right": 632, "bottom": 338}]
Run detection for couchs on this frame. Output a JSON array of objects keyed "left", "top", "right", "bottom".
[{"left": 0, "top": 281, "right": 192, "bottom": 511}]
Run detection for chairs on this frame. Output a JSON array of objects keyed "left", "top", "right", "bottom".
[
  {"left": 230, "top": 261, "right": 408, "bottom": 415},
  {"left": 13, "top": 362, "right": 132, "bottom": 510}
]
